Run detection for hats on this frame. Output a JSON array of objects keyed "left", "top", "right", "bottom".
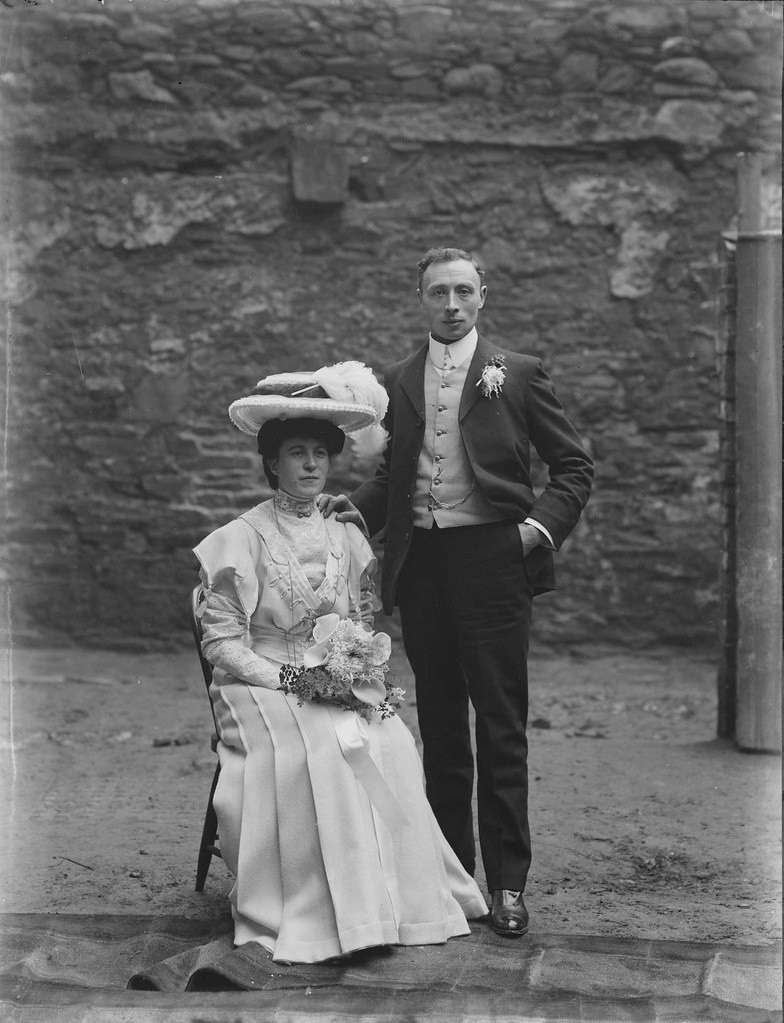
[{"left": 228, "top": 360, "right": 390, "bottom": 436}]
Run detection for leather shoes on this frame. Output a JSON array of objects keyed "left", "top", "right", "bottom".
[{"left": 491, "top": 889, "right": 528, "bottom": 935}]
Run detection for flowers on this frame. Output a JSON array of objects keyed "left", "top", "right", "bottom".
[
  {"left": 277, "top": 613, "right": 407, "bottom": 721},
  {"left": 474, "top": 354, "right": 509, "bottom": 401}
]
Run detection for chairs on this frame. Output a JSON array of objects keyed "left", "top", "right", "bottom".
[{"left": 183, "top": 585, "right": 225, "bottom": 893}]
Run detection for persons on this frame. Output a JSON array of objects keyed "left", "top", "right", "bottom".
[
  {"left": 317, "top": 248, "right": 594, "bottom": 936},
  {"left": 191, "top": 361, "right": 489, "bottom": 963}
]
